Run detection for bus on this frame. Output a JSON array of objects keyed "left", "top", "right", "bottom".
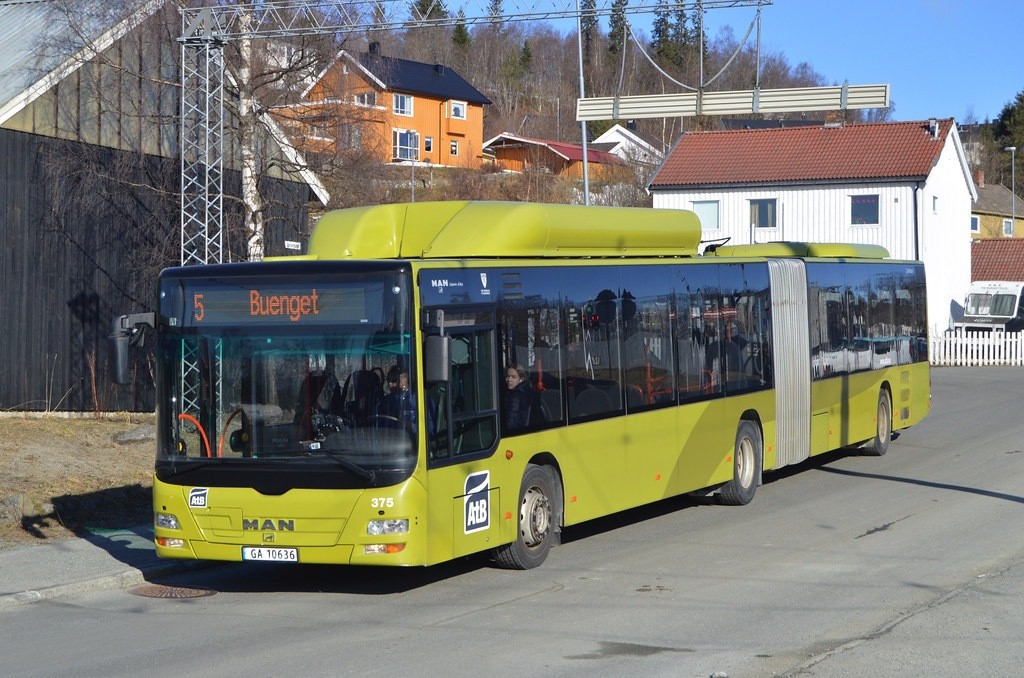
[{"left": 108, "top": 201, "right": 932, "bottom": 570}]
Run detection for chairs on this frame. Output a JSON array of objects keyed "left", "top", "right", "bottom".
[
  {"left": 543, "top": 340, "right": 745, "bottom": 425},
  {"left": 290, "top": 370, "right": 383, "bottom": 430}
]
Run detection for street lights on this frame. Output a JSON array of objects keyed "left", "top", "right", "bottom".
[{"left": 1004, "top": 146, "right": 1016, "bottom": 238}]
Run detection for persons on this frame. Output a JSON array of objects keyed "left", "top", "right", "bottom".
[
  {"left": 376, "top": 366, "right": 434, "bottom": 441},
  {"left": 502, "top": 363, "right": 537, "bottom": 431}
]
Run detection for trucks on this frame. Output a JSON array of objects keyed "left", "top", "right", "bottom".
[{"left": 954, "top": 281, "right": 1024, "bottom": 360}]
